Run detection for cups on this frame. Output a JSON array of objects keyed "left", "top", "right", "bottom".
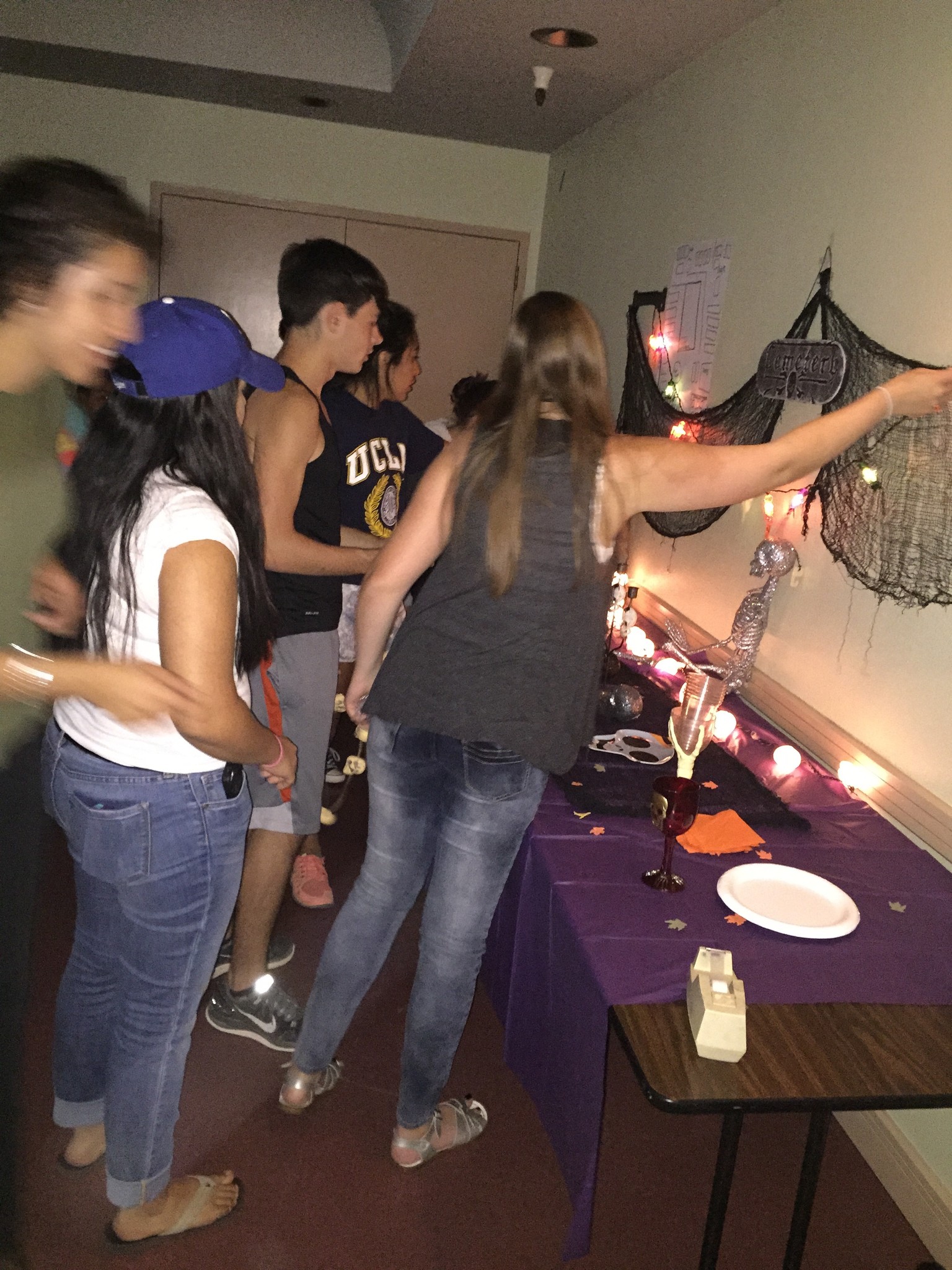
[{"left": 669, "top": 674, "right": 728, "bottom": 762}]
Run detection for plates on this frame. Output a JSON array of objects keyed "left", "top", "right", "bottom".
[{"left": 717, "top": 863, "right": 861, "bottom": 939}]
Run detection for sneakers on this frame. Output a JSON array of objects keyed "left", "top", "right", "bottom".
[
  {"left": 325, "top": 745, "right": 345, "bottom": 783},
  {"left": 204, "top": 980, "right": 306, "bottom": 1050},
  {"left": 292, "top": 856, "right": 333, "bottom": 909},
  {"left": 210, "top": 931, "right": 296, "bottom": 979}
]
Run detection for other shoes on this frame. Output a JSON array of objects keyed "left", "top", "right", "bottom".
[{"left": 320, "top": 806, "right": 338, "bottom": 826}]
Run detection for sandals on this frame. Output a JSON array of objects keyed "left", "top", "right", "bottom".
[
  {"left": 277, "top": 1057, "right": 346, "bottom": 1114},
  {"left": 393, "top": 1094, "right": 487, "bottom": 1172}
]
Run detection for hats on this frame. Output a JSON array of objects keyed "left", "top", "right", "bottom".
[{"left": 106, "top": 294, "right": 287, "bottom": 401}]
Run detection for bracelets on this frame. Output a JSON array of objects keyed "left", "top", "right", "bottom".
[
  {"left": 260, "top": 733, "right": 282, "bottom": 767},
  {"left": 874, "top": 385, "right": 894, "bottom": 419}
]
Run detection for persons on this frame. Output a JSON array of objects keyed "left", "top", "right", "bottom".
[
  {"left": 202, "top": 236, "right": 390, "bottom": 1055},
  {"left": 276, "top": 291, "right": 951, "bottom": 1172},
  {"left": 0, "top": 155, "right": 196, "bottom": 1270},
  {"left": 291, "top": 299, "right": 452, "bottom": 908},
  {"left": 423, "top": 373, "right": 498, "bottom": 442},
  {"left": 39, "top": 294, "right": 300, "bottom": 1248}
]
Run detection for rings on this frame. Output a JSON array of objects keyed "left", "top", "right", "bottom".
[{"left": 934, "top": 405, "right": 941, "bottom": 412}]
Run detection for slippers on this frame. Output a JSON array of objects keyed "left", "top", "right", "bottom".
[
  {"left": 53, "top": 1145, "right": 107, "bottom": 1175},
  {"left": 102, "top": 1174, "right": 245, "bottom": 1251}
]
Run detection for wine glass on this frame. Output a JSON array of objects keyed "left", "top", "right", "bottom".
[{"left": 641, "top": 777, "right": 700, "bottom": 892}]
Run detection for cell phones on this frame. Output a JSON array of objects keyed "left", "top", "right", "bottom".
[{"left": 221, "top": 755, "right": 246, "bottom": 799}]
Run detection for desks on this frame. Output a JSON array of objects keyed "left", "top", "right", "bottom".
[{"left": 481, "top": 604, "right": 952, "bottom": 1269}]
